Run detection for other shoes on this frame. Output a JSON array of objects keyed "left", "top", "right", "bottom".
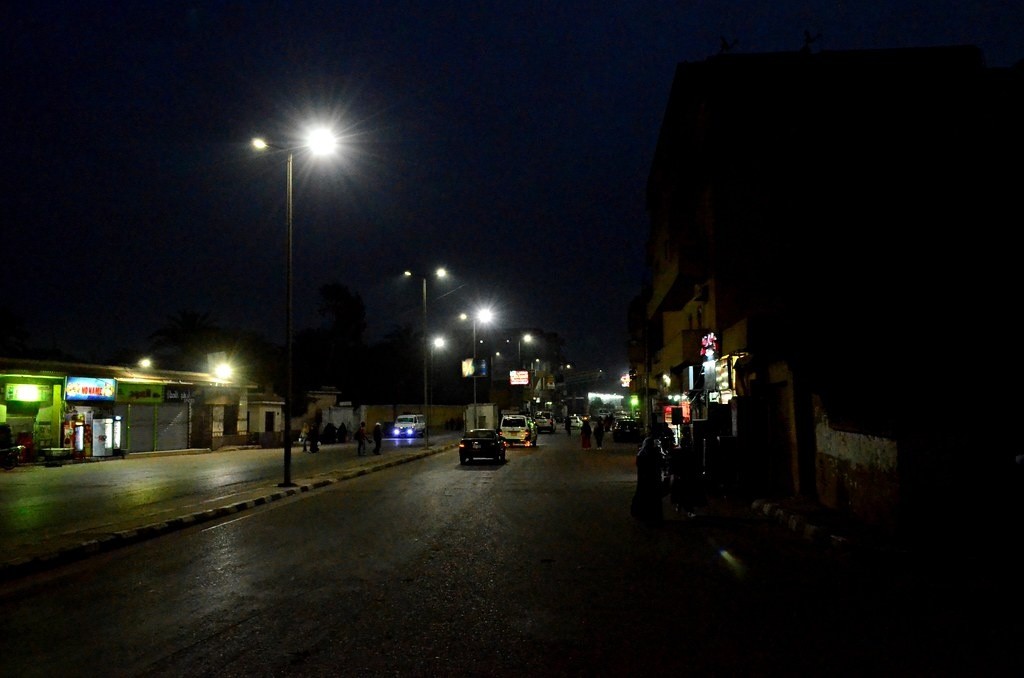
[
  {"left": 596, "top": 447, "right": 602, "bottom": 450},
  {"left": 583, "top": 448, "right": 590, "bottom": 451}
]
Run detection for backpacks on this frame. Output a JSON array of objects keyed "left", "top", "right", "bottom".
[{"left": 354, "top": 430, "right": 361, "bottom": 441}]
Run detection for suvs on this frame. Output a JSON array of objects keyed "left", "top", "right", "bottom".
[
  {"left": 499, "top": 415, "right": 539, "bottom": 447},
  {"left": 535, "top": 411, "right": 557, "bottom": 434}
]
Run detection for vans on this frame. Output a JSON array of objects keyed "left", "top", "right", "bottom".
[{"left": 392, "top": 414, "right": 425, "bottom": 438}]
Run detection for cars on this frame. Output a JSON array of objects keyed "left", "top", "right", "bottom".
[
  {"left": 594, "top": 409, "right": 611, "bottom": 418},
  {"left": 613, "top": 417, "right": 640, "bottom": 443},
  {"left": 459, "top": 428, "right": 507, "bottom": 465},
  {"left": 569, "top": 416, "right": 584, "bottom": 429}
]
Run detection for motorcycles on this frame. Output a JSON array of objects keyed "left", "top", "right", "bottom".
[
  {"left": 635, "top": 425, "right": 709, "bottom": 518},
  {"left": 0, "top": 444, "right": 26, "bottom": 470}
]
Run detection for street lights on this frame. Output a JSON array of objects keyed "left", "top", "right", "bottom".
[
  {"left": 457, "top": 300, "right": 502, "bottom": 429},
  {"left": 428, "top": 334, "right": 449, "bottom": 435},
  {"left": 403, "top": 266, "right": 449, "bottom": 448},
  {"left": 630, "top": 394, "right": 640, "bottom": 418},
  {"left": 249, "top": 110, "right": 358, "bottom": 488},
  {"left": 518, "top": 333, "right": 536, "bottom": 371}
]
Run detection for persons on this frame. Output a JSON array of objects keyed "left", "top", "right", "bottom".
[
  {"left": 593, "top": 419, "right": 605, "bottom": 451},
  {"left": 372, "top": 422, "right": 382, "bottom": 455},
  {"left": 299, "top": 422, "right": 320, "bottom": 453},
  {"left": 680, "top": 426, "right": 692, "bottom": 447},
  {"left": 635, "top": 438, "right": 665, "bottom": 534},
  {"left": 590, "top": 412, "right": 616, "bottom": 432},
  {"left": 354, "top": 421, "right": 372, "bottom": 456},
  {"left": 347, "top": 421, "right": 355, "bottom": 442},
  {"left": 662, "top": 422, "right": 676, "bottom": 451},
  {"left": 564, "top": 416, "right": 572, "bottom": 437},
  {"left": 580, "top": 421, "right": 592, "bottom": 451}
]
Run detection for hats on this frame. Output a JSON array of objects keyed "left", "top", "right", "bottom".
[{"left": 376, "top": 423, "right": 381, "bottom": 425}]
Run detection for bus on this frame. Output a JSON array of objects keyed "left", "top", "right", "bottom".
[{"left": 615, "top": 411, "right": 627, "bottom": 417}]
